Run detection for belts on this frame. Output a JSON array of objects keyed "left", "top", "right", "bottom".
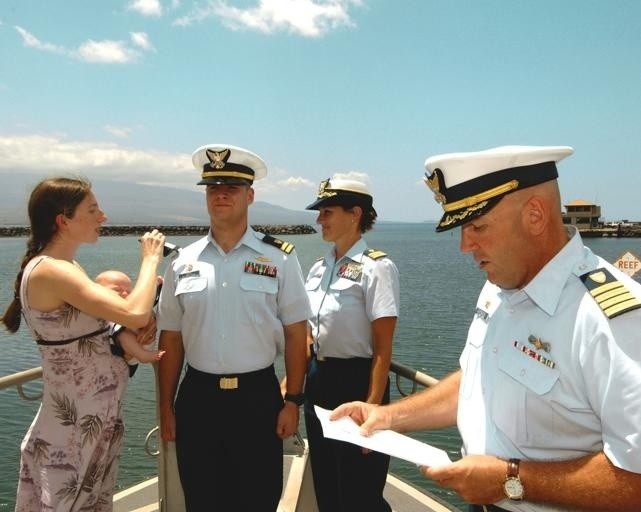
[{"left": 215, "top": 376, "right": 242, "bottom": 390}]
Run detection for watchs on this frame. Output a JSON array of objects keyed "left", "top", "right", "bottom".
[
  {"left": 282, "top": 390, "right": 308, "bottom": 408},
  {"left": 504, "top": 455, "right": 529, "bottom": 502}
]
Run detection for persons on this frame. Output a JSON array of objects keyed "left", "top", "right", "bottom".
[
  {"left": 279, "top": 173, "right": 400, "bottom": 512},
  {"left": 152, "top": 143, "right": 309, "bottom": 511},
  {"left": 90, "top": 269, "right": 168, "bottom": 381},
  {"left": 330, "top": 143, "right": 641, "bottom": 511},
  {"left": 17, "top": 177, "right": 166, "bottom": 510}
]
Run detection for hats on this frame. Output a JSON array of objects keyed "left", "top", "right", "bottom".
[
  {"left": 425, "top": 146, "right": 573, "bottom": 232},
  {"left": 305, "top": 174, "right": 372, "bottom": 210},
  {"left": 192, "top": 144, "right": 268, "bottom": 185}
]
